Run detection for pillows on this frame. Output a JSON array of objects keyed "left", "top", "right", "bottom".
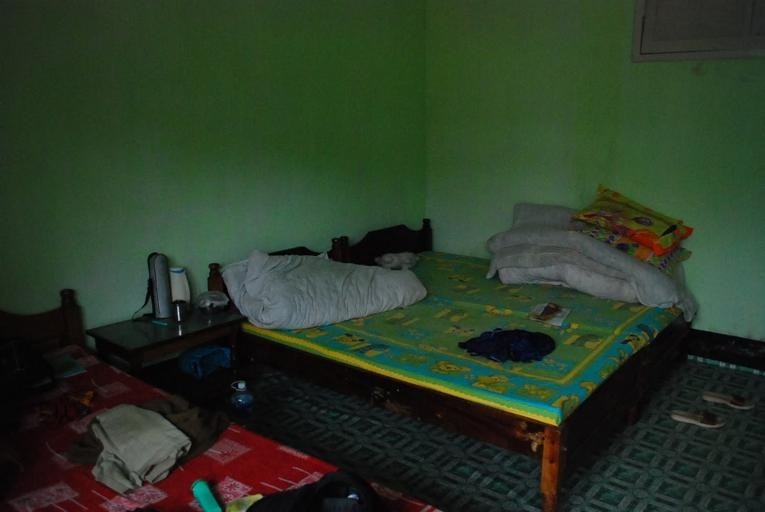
[{"left": 570, "top": 184, "right": 693, "bottom": 256}]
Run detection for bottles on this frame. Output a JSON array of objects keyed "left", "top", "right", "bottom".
[{"left": 206, "top": 261, "right": 225, "bottom": 291}]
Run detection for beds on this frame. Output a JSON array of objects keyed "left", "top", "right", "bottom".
[
  {"left": 234, "top": 218, "right": 693, "bottom": 506},
  {"left": 2, "top": 289, "right": 448, "bottom": 512}
]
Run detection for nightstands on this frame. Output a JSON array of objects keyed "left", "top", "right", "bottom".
[{"left": 86, "top": 311, "right": 247, "bottom": 402}]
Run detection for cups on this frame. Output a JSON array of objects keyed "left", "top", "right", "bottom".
[{"left": 172, "top": 298, "right": 189, "bottom": 323}]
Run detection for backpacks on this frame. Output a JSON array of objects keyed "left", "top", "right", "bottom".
[{"left": 457, "top": 328, "right": 557, "bottom": 363}]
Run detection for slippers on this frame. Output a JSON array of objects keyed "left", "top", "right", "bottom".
[
  {"left": 702, "top": 389, "right": 757, "bottom": 411},
  {"left": 668, "top": 408, "right": 727, "bottom": 429}
]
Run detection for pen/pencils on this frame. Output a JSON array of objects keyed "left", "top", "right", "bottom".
[{"left": 151, "top": 320, "right": 168, "bottom": 326}]
